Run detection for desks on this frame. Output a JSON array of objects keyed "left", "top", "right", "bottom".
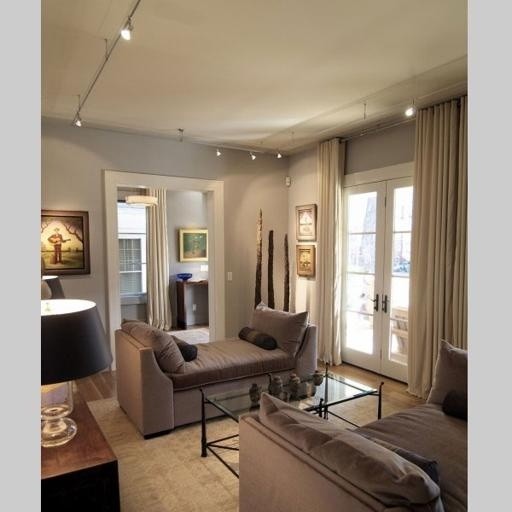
[{"left": 41, "top": 392, "right": 120, "bottom": 511}]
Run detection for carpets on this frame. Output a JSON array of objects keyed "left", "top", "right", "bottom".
[{"left": 86, "top": 369, "right": 411, "bottom": 512}]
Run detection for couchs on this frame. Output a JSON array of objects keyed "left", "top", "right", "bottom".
[
  {"left": 239, "top": 340, "right": 467, "bottom": 512},
  {"left": 115, "top": 304, "right": 317, "bottom": 438}
]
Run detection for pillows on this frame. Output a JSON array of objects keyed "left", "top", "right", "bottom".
[
  {"left": 427, "top": 340, "right": 467, "bottom": 413},
  {"left": 252, "top": 302, "right": 309, "bottom": 356},
  {"left": 121, "top": 319, "right": 184, "bottom": 374},
  {"left": 259, "top": 394, "right": 444, "bottom": 511}
]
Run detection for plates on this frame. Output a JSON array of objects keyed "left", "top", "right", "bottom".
[{"left": 177, "top": 273, "right": 192, "bottom": 281}]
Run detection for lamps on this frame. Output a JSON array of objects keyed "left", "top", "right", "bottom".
[
  {"left": 125, "top": 189, "right": 158, "bottom": 207},
  {"left": 276, "top": 151, "right": 281, "bottom": 159},
  {"left": 41, "top": 299, "right": 112, "bottom": 448},
  {"left": 74, "top": 95, "right": 83, "bottom": 127},
  {"left": 120, "top": 19, "right": 134, "bottom": 40},
  {"left": 250, "top": 151, "right": 256, "bottom": 160},
  {"left": 405, "top": 98, "right": 417, "bottom": 115},
  {"left": 216, "top": 146, "right": 220, "bottom": 157}
]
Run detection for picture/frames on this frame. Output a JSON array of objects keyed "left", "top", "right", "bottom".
[
  {"left": 296, "top": 244, "right": 315, "bottom": 276},
  {"left": 41, "top": 209, "right": 90, "bottom": 275},
  {"left": 295, "top": 204, "right": 317, "bottom": 240},
  {"left": 179, "top": 228, "right": 208, "bottom": 262}
]
